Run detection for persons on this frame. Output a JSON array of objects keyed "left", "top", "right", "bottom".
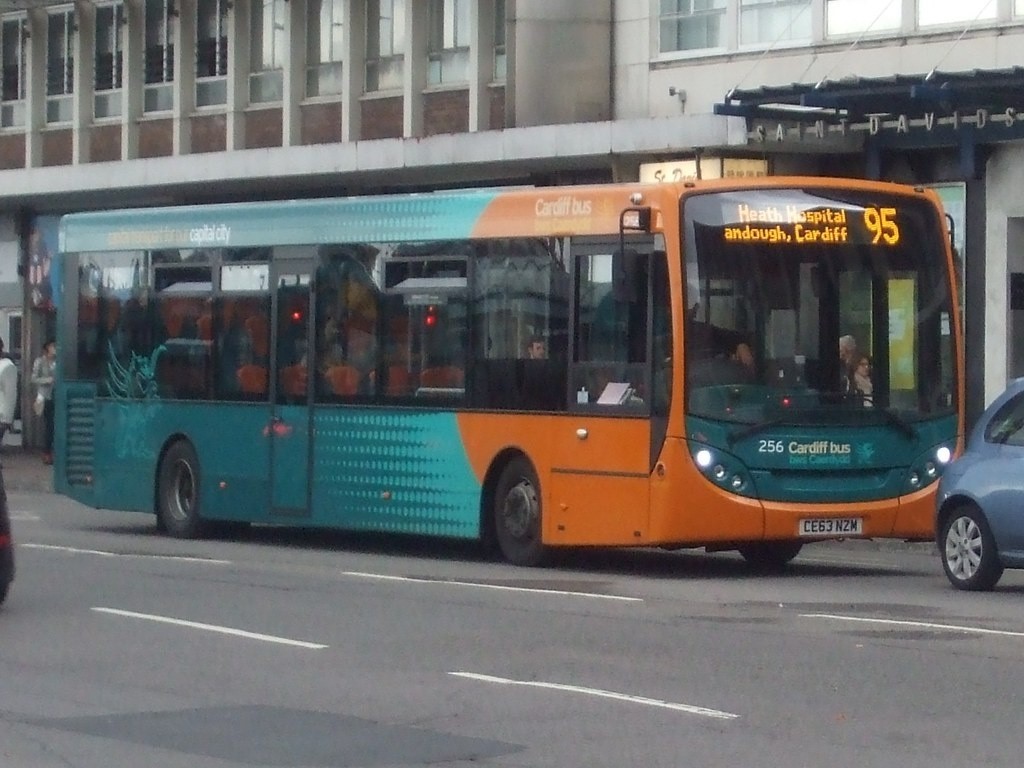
[
  {"left": 840, "top": 350, "right": 874, "bottom": 407},
  {"left": 525, "top": 335, "right": 546, "bottom": 358},
  {"left": 584, "top": 253, "right": 756, "bottom": 403},
  {"left": 840, "top": 335, "right": 857, "bottom": 364},
  {"left": 300, "top": 311, "right": 344, "bottom": 367},
  {"left": 0, "top": 336, "right": 56, "bottom": 464}
]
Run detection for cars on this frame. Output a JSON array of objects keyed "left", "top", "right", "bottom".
[{"left": 932, "top": 375, "right": 1023, "bottom": 592}]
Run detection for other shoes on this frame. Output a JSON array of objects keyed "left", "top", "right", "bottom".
[{"left": 42, "top": 454, "right": 53, "bottom": 465}]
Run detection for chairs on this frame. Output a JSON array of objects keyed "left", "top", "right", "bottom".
[{"left": 82, "top": 293, "right": 466, "bottom": 399}]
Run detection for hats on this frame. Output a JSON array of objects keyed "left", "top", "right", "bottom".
[{"left": 43, "top": 336, "right": 57, "bottom": 350}]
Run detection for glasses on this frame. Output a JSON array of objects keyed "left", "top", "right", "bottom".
[{"left": 859, "top": 363, "right": 873, "bottom": 370}]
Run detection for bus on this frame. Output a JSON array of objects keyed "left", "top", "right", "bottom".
[{"left": 51, "top": 175, "right": 967, "bottom": 569}]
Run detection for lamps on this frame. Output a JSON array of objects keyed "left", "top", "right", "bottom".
[{"left": 668, "top": 87, "right": 686, "bottom": 103}]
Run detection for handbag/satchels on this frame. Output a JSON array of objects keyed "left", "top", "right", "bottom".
[{"left": 31, "top": 394, "right": 45, "bottom": 417}]
